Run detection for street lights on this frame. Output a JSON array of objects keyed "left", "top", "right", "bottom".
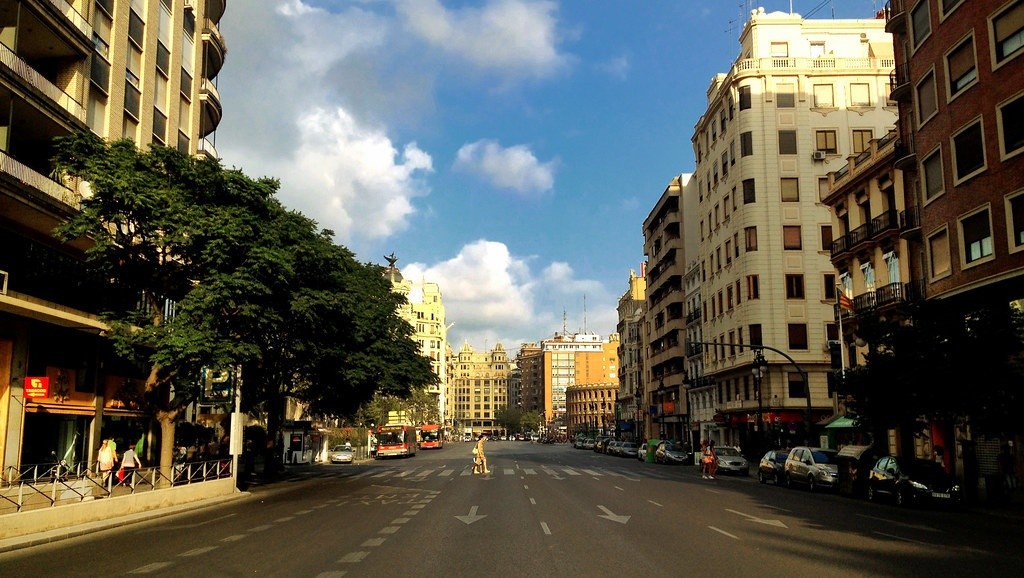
[
  {"left": 751, "top": 350, "right": 769, "bottom": 451},
  {"left": 658, "top": 382, "right": 669, "bottom": 442}
]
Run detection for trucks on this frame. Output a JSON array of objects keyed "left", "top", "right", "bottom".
[{"left": 462, "top": 427, "right": 472, "bottom": 443}]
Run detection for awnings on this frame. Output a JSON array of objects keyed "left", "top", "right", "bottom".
[
  {"left": 825, "top": 411, "right": 874, "bottom": 429},
  {"left": 816, "top": 413, "right": 841, "bottom": 425}
]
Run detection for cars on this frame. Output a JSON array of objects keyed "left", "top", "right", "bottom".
[
  {"left": 475, "top": 431, "right": 568, "bottom": 445},
  {"left": 655, "top": 442, "right": 691, "bottom": 466},
  {"left": 637, "top": 444, "right": 648, "bottom": 462},
  {"left": 758, "top": 449, "right": 790, "bottom": 487},
  {"left": 868, "top": 455, "right": 964, "bottom": 511},
  {"left": 573, "top": 436, "right": 639, "bottom": 458},
  {"left": 331, "top": 444, "right": 355, "bottom": 464},
  {"left": 699, "top": 445, "right": 750, "bottom": 476}
]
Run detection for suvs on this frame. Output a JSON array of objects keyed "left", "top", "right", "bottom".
[{"left": 782, "top": 446, "right": 843, "bottom": 493}]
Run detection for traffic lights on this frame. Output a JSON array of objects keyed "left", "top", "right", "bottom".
[
  {"left": 694, "top": 342, "right": 702, "bottom": 354},
  {"left": 687, "top": 342, "right": 693, "bottom": 358}
]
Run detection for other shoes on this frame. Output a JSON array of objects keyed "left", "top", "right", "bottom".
[
  {"left": 484, "top": 470, "right": 490, "bottom": 474},
  {"left": 474, "top": 471, "right": 480, "bottom": 474},
  {"left": 709, "top": 476, "right": 714, "bottom": 479},
  {"left": 702, "top": 476, "right": 708, "bottom": 479}
]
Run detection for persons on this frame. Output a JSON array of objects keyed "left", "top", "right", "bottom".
[
  {"left": 345, "top": 438, "right": 352, "bottom": 450},
  {"left": 96, "top": 437, "right": 117, "bottom": 498},
  {"left": 121, "top": 444, "right": 142, "bottom": 494},
  {"left": 701, "top": 439, "right": 714, "bottom": 479},
  {"left": 709, "top": 440, "right": 719, "bottom": 479},
  {"left": 472, "top": 435, "right": 494, "bottom": 474},
  {"left": 170, "top": 437, "right": 232, "bottom": 478},
  {"left": 997, "top": 444, "right": 1019, "bottom": 491},
  {"left": 723, "top": 441, "right": 730, "bottom": 447},
  {"left": 780, "top": 442, "right": 793, "bottom": 455},
  {"left": 732, "top": 441, "right": 741, "bottom": 454}
]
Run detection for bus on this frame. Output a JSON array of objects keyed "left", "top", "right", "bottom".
[
  {"left": 418, "top": 424, "right": 443, "bottom": 450},
  {"left": 376, "top": 424, "right": 416, "bottom": 460}
]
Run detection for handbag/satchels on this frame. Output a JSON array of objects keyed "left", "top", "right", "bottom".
[{"left": 472, "top": 448, "right": 478, "bottom": 454}]
